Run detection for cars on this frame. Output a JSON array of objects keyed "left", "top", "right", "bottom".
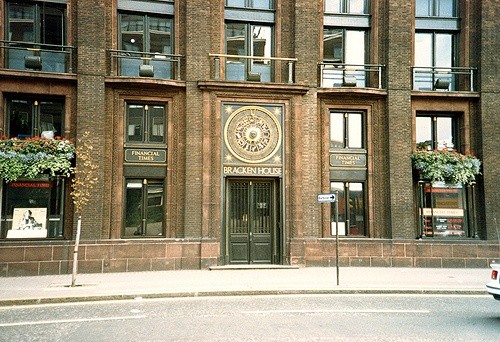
[{"left": 485, "top": 260, "right": 500, "bottom": 301}]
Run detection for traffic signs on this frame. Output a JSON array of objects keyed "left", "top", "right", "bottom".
[{"left": 317, "top": 193, "right": 336, "bottom": 203}]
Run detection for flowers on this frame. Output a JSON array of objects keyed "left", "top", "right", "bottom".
[
  {"left": 411, "top": 145, "right": 483, "bottom": 188},
  {"left": 0, "top": 134, "right": 77, "bottom": 183}
]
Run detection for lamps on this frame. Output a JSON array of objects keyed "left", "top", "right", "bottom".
[
  {"left": 245, "top": 71, "right": 260, "bottom": 81},
  {"left": 138, "top": 64, "right": 153, "bottom": 77},
  {"left": 343, "top": 75, "right": 356, "bottom": 86},
  {"left": 23, "top": 55, "right": 44, "bottom": 70},
  {"left": 434, "top": 77, "right": 451, "bottom": 92}
]
